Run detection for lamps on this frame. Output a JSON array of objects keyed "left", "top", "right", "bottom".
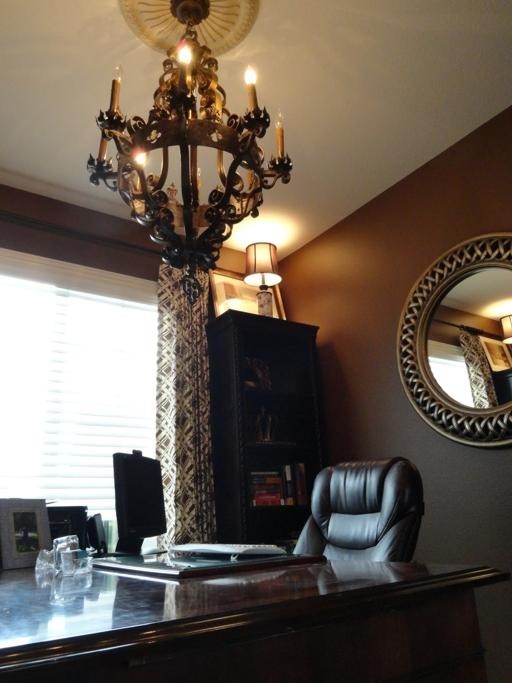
[
  {"left": 70, "top": 0, "right": 295, "bottom": 301},
  {"left": 242, "top": 243, "right": 283, "bottom": 317}
]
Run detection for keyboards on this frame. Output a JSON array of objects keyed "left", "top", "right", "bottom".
[{"left": 170, "top": 542, "right": 288, "bottom": 561}]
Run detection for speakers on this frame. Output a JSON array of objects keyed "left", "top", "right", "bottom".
[{"left": 86, "top": 513, "right": 106, "bottom": 550}]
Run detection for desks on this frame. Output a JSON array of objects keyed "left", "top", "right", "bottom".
[{"left": 0, "top": 540, "right": 511, "bottom": 682}]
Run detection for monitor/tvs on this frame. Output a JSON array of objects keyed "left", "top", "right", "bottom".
[{"left": 107, "top": 449, "right": 168, "bottom": 557}]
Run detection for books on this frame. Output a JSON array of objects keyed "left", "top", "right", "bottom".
[{"left": 246, "top": 461, "right": 313, "bottom": 506}]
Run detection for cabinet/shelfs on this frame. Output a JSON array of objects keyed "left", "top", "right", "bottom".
[{"left": 200, "top": 309, "right": 327, "bottom": 544}]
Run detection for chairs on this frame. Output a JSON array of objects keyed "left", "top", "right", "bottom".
[{"left": 293, "top": 454, "right": 431, "bottom": 562}]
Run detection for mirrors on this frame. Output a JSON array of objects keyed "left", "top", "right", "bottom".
[{"left": 397, "top": 234, "right": 511, "bottom": 449}]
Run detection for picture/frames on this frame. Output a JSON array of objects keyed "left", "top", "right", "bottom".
[
  {"left": 208, "top": 267, "right": 287, "bottom": 319},
  {"left": 3, "top": 499, "right": 53, "bottom": 568}
]
